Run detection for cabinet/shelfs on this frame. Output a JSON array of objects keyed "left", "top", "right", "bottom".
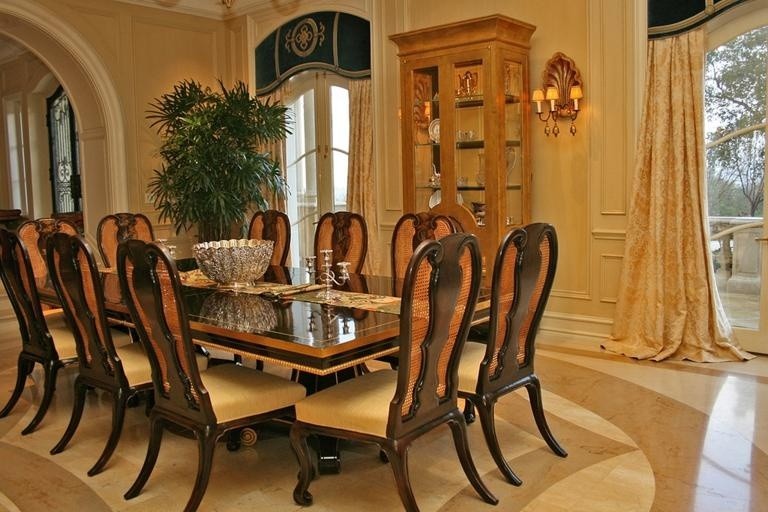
[{"left": 388, "top": 12, "right": 534, "bottom": 319}]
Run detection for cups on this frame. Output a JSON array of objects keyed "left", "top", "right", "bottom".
[
  {"left": 455, "top": 128, "right": 476, "bottom": 141},
  {"left": 505, "top": 216, "right": 514, "bottom": 224}
]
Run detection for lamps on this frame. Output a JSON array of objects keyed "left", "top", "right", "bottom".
[{"left": 531, "top": 49, "right": 585, "bottom": 138}]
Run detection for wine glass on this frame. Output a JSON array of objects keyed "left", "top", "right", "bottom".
[{"left": 471, "top": 200, "right": 487, "bottom": 226}]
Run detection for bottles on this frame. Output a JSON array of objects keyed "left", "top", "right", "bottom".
[{"left": 156, "top": 238, "right": 177, "bottom": 263}]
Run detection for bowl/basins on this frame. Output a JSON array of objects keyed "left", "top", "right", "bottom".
[
  {"left": 190, "top": 238, "right": 276, "bottom": 289},
  {"left": 195, "top": 289, "right": 279, "bottom": 332}
]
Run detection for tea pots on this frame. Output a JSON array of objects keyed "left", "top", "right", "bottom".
[{"left": 457, "top": 70, "right": 479, "bottom": 95}]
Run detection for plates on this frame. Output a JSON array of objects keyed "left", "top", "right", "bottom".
[
  {"left": 428, "top": 118, "right": 441, "bottom": 144},
  {"left": 427, "top": 189, "right": 464, "bottom": 210},
  {"left": 505, "top": 146, "right": 517, "bottom": 176}
]
